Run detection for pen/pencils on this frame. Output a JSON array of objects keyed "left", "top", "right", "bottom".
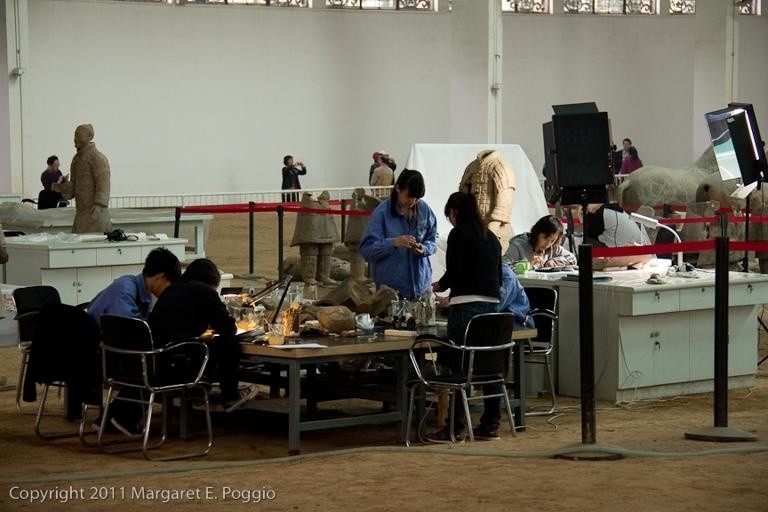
[{"left": 284, "top": 306, "right": 301, "bottom": 336}]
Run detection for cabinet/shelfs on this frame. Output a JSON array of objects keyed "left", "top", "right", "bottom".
[
  {"left": 1, "top": 237, "right": 186, "bottom": 306},
  {"left": 556, "top": 267, "right": 768, "bottom": 404}
]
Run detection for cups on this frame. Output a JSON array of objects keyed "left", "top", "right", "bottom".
[
  {"left": 267, "top": 324, "right": 285, "bottom": 345},
  {"left": 515, "top": 260, "right": 530, "bottom": 276}
]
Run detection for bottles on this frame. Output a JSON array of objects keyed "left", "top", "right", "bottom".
[{"left": 392, "top": 315, "right": 408, "bottom": 330}]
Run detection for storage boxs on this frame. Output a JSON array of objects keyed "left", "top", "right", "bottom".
[{"left": 0, "top": 283, "right": 28, "bottom": 348}]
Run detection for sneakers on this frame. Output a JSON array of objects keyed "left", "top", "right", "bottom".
[
  {"left": 423, "top": 429, "right": 465, "bottom": 445},
  {"left": 110, "top": 414, "right": 145, "bottom": 440},
  {"left": 91, "top": 417, "right": 121, "bottom": 435},
  {"left": 464, "top": 424, "right": 500, "bottom": 441},
  {"left": 222, "top": 385, "right": 258, "bottom": 414}
]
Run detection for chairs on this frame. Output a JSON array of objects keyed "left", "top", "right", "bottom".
[
  {"left": 10, "top": 284, "right": 214, "bottom": 461},
  {"left": 404, "top": 284, "right": 559, "bottom": 448}
]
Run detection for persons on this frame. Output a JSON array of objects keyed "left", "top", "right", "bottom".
[
  {"left": 357, "top": 137, "right": 657, "bottom": 444},
  {"left": 281, "top": 155, "right": 307, "bottom": 202},
  {"left": 344, "top": 188, "right": 384, "bottom": 283},
  {"left": 38, "top": 174, "right": 66, "bottom": 209},
  {"left": 51, "top": 124, "right": 113, "bottom": 233},
  {"left": 86, "top": 247, "right": 182, "bottom": 434},
  {"left": 40, "top": 155, "right": 70, "bottom": 189},
  {"left": 143, "top": 258, "right": 259, "bottom": 412},
  {"left": 290, "top": 191, "right": 343, "bottom": 288}
]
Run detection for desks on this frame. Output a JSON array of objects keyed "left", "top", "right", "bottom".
[
  {"left": 173, "top": 323, "right": 538, "bottom": 456},
  {"left": 0, "top": 206, "right": 214, "bottom": 259},
  {"left": 514, "top": 265, "right": 603, "bottom": 394}
]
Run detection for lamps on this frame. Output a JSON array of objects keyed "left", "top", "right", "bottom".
[{"left": 628, "top": 212, "right": 684, "bottom": 270}]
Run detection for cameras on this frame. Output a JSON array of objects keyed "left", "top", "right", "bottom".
[{"left": 104, "top": 229, "right": 126, "bottom": 240}]
